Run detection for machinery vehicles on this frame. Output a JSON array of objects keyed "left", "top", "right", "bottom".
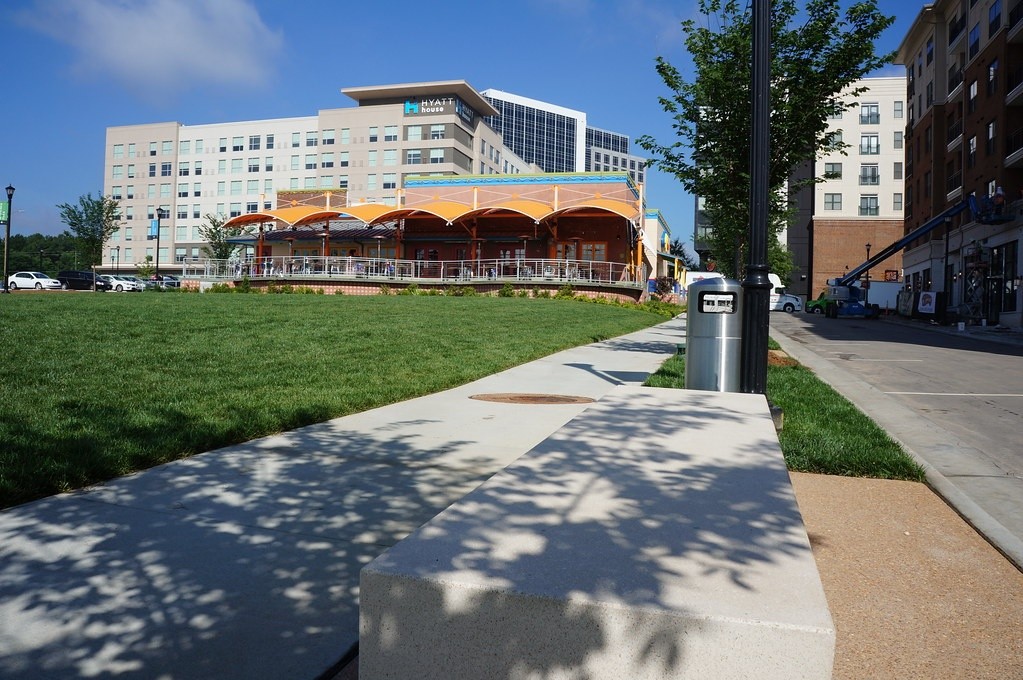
[{"left": 824, "top": 188, "right": 1017, "bottom": 320}]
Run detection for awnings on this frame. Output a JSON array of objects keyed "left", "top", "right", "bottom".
[{"left": 218, "top": 199, "right": 638, "bottom": 280}]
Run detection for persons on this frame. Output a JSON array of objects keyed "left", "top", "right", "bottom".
[
  {"left": 158, "top": 273, "right": 163, "bottom": 284},
  {"left": 151, "top": 273, "right": 158, "bottom": 281}
]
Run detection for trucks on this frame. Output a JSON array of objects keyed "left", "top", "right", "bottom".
[
  {"left": 767, "top": 273, "right": 802, "bottom": 313},
  {"left": 805, "top": 279, "right": 903, "bottom": 315}
]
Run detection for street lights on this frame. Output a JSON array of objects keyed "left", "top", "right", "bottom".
[
  {"left": 1, "top": 183, "right": 16, "bottom": 293},
  {"left": 155, "top": 206, "right": 164, "bottom": 278},
  {"left": 865, "top": 242, "right": 871, "bottom": 303}
]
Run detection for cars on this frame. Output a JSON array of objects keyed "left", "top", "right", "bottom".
[
  {"left": 99, "top": 274, "right": 180, "bottom": 292},
  {"left": 8, "top": 271, "right": 62, "bottom": 290}
]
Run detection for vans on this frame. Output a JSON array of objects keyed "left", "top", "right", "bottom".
[{"left": 56, "top": 270, "right": 113, "bottom": 292}]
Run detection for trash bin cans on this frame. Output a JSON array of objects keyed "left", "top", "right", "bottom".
[{"left": 685, "top": 276, "right": 744, "bottom": 394}]
